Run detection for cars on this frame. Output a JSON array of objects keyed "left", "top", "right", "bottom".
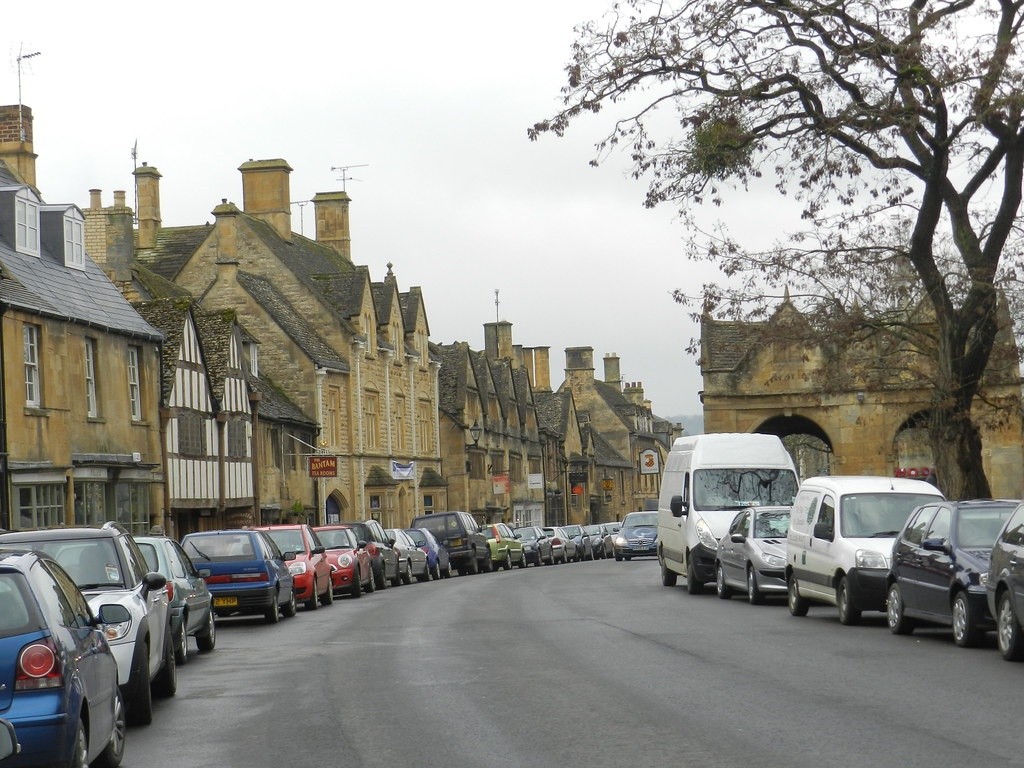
[
  {"left": 0, "top": 549, "right": 132, "bottom": 768},
  {"left": 714, "top": 506, "right": 794, "bottom": 604},
  {"left": 478, "top": 522, "right": 623, "bottom": 570},
  {"left": 613, "top": 511, "right": 661, "bottom": 561},
  {"left": 181, "top": 519, "right": 452, "bottom": 623}
]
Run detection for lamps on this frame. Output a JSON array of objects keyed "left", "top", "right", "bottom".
[{"left": 464, "top": 418, "right": 485, "bottom": 450}]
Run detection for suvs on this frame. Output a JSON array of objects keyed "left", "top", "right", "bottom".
[
  {"left": 410, "top": 511, "right": 493, "bottom": 574},
  {"left": 55, "top": 535, "right": 216, "bottom": 665},
  {"left": 987, "top": 502, "right": 1024, "bottom": 660},
  {"left": 886, "top": 498, "right": 1024, "bottom": 646},
  {"left": 0, "top": 521, "right": 177, "bottom": 725}
]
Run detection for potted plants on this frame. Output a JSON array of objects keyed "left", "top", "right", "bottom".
[{"left": 285, "top": 512, "right": 305, "bottom": 524}]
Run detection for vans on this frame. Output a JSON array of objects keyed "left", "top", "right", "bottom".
[
  {"left": 784, "top": 476, "right": 946, "bottom": 625},
  {"left": 657, "top": 432, "right": 800, "bottom": 594}
]
